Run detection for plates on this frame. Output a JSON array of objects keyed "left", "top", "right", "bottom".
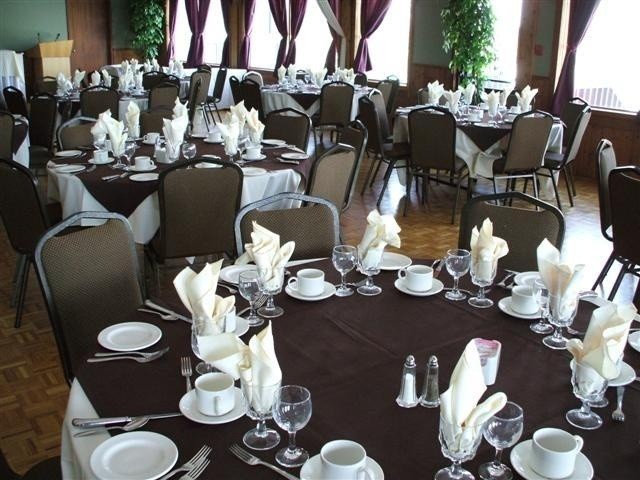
[
  {"left": 569, "top": 356, "right": 636, "bottom": 387},
  {"left": 52, "top": 133, "right": 307, "bottom": 184},
  {"left": 380, "top": 252, "right": 412, "bottom": 271},
  {"left": 96, "top": 321, "right": 164, "bottom": 352},
  {"left": 234, "top": 317, "right": 250, "bottom": 337},
  {"left": 628, "top": 330, "right": 640, "bottom": 353},
  {"left": 509, "top": 438, "right": 594, "bottom": 480},
  {"left": 394, "top": 278, "right": 444, "bottom": 295},
  {"left": 90, "top": 432, "right": 177, "bottom": 480},
  {"left": 285, "top": 281, "right": 337, "bottom": 301},
  {"left": 497, "top": 295, "right": 547, "bottom": 319},
  {"left": 415, "top": 103, "right": 534, "bottom": 128},
  {"left": 513, "top": 271, "right": 549, "bottom": 289},
  {"left": 300, "top": 454, "right": 385, "bottom": 480},
  {"left": 219, "top": 264, "right": 261, "bottom": 285},
  {"left": 180, "top": 387, "right": 248, "bottom": 426}
]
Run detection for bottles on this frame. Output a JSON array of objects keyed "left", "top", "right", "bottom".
[
  {"left": 419, "top": 355, "right": 442, "bottom": 409},
  {"left": 394, "top": 355, "right": 419, "bottom": 407}
]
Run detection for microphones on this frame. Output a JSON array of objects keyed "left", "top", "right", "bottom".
[
  {"left": 37, "top": 32, "right": 40, "bottom": 43},
  {"left": 54, "top": 33, "right": 61, "bottom": 41}
]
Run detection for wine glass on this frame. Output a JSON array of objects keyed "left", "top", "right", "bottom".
[
  {"left": 191, "top": 318, "right": 223, "bottom": 374},
  {"left": 356, "top": 244, "right": 382, "bottom": 296},
  {"left": 239, "top": 267, "right": 284, "bottom": 328},
  {"left": 434, "top": 409, "right": 481, "bottom": 480},
  {"left": 242, "top": 381, "right": 281, "bottom": 451},
  {"left": 566, "top": 360, "right": 609, "bottom": 430},
  {"left": 279, "top": 74, "right": 369, "bottom": 94},
  {"left": 530, "top": 280, "right": 578, "bottom": 350},
  {"left": 444, "top": 249, "right": 472, "bottom": 301},
  {"left": 274, "top": 385, "right": 313, "bottom": 468},
  {"left": 332, "top": 244, "right": 357, "bottom": 298},
  {"left": 57, "top": 80, "right": 145, "bottom": 102},
  {"left": 478, "top": 400, "right": 524, "bottom": 480},
  {"left": 468, "top": 252, "right": 496, "bottom": 309}
]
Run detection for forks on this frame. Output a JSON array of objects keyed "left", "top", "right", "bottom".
[
  {"left": 226, "top": 443, "right": 299, "bottom": 480},
  {"left": 235, "top": 292, "right": 269, "bottom": 316},
  {"left": 496, "top": 272, "right": 516, "bottom": 291},
  {"left": 176, "top": 458, "right": 211, "bottom": 480},
  {"left": 88, "top": 346, "right": 171, "bottom": 365},
  {"left": 335, "top": 277, "right": 369, "bottom": 287},
  {"left": 443, "top": 286, "right": 491, "bottom": 297},
  {"left": 161, "top": 445, "right": 212, "bottom": 480},
  {"left": 611, "top": 386, "right": 627, "bottom": 422},
  {"left": 565, "top": 324, "right": 640, "bottom": 337},
  {"left": 182, "top": 356, "right": 193, "bottom": 392}
]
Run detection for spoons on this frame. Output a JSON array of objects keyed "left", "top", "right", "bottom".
[
  {"left": 216, "top": 281, "right": 238, "bottom": 293},
  {"left": 138, "top": 307, "right": 178, "bottom": 321},
  {"left": 76, "top": 414, "right": 152, "bottom": 440}
]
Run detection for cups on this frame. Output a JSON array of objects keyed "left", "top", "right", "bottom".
[
  {"left": 531, "top": 428, "right": 584, "bottom": 480},
  {"left": 320, "top": 439, "right": 374, "bottom": 480},
  {"left": 196, "top": 374, "right": 235, "bottom": 416},
  {"left": 510, "top": 286, "right": 542, "bottom": 315},
  {"left": 225, "top": 306, "right": 236, "bottom": 333},
  {"left": 398, "top": 265, "right": 433, "bottom": 291},
  {"left": 288, "top": 267, "right": 325, "bottom": 296}
]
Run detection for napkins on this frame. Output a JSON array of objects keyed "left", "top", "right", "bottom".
[
  {"left": 352, "top": 209, "right": 401, "bottom": 272},
  {"left": 170, "top": 257, "right": 239, "bottom": 356},
  {"left": 564, "top": 295, "right": 637, "bottom": 390},
  {"left": 436, "top": 336, "right": 513, "bottom": 459},
  {"left": 466, "top": 217, "right": 509, "bottom": 280},
  {"left": 533, "top": 233, "right": 586, "bottom": 326},
  {"left": 199, "top": 321, "right": 284, "bottom": 423},
  {"left": 240, "top": 217, "right": 295, "bottom": 296}
]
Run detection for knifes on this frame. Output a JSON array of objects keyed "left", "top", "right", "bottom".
[
  {"left": 145, "top": 299, "right": 193, "bottom": 324},
  {"left": 72, "top": 412, "right": 185, "bottom": 427},
  {"left": 433, "top": 256, "right": 446, "bottom": 279}
]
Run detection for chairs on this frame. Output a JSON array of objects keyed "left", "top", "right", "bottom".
[
  {"left": 233, "top": 188, "right": 342, "bottom": 264},
  {"left": 606, "top": 161, "right": 640, "bottom": 301},
  {"left": 1, "top": 61, "right": 595, "bottom": 226},
  {"left": 1, "top": 444, "right": 64, "bottom": 480},
  {"left": 149, "top": 158, "right": 247, "bottom": 312},
  {"left": 31, "top": 208, "right": 148, "bottom": 393},
  {"left": 456, "top": 190, "right": 571, "bottom": 278},
  {"left": 588, "top": 136, "right": 618, "bottom": 294},
  {"left": 3, "top": 157, "right": 88, "bottom": 330}
]
